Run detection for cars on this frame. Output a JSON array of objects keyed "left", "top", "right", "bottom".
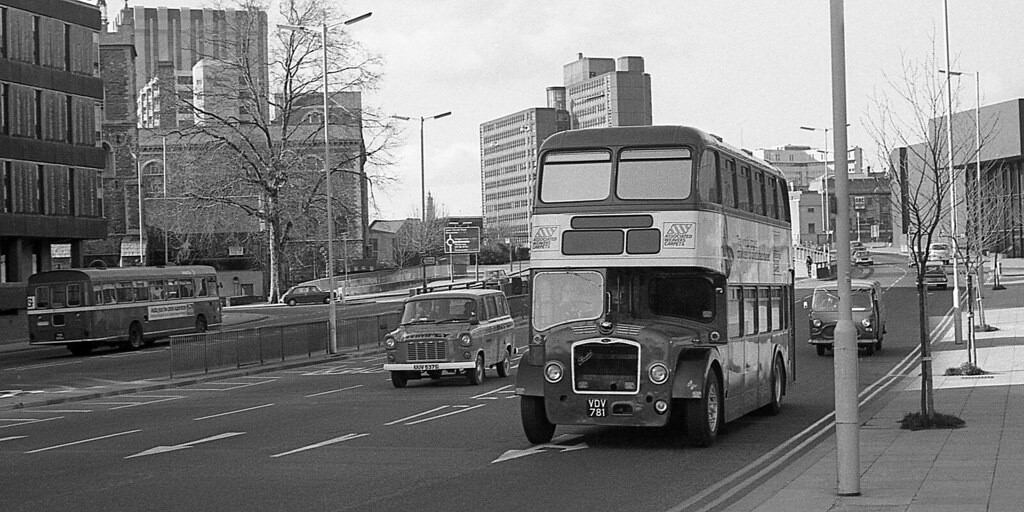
[
  {"left": 481, "top": 269, "right": 509, "bottom": 285},
  {"left": 907, "top": 243, "right": 951, "bottom": 290},
  {"left": 849, "top": 241, "right": 875, "bottom": 268},
  {"left": 280, "top": 284, "right": 338, "bottom": 306}
]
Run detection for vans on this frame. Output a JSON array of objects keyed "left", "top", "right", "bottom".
[{"left": 800, "top": 279, "right": 887, "bottom": 356}]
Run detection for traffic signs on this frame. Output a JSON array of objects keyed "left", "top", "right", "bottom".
[
  {"left": 420, "top": 256, "right": 436, "bottom": 266},
  {"left": 443, "top": 227, "right": 481, "bottom": 254}
]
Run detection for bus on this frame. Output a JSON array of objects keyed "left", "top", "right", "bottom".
[
  {"left": 25, "top": 258, "right": 223, "bottom": 356},
  {"left": 513, "top": 124, "right": 797, "bottom": 450}
]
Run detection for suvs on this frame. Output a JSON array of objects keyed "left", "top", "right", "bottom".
[{"left": 382, "top": 288, "right": 519, "bottom": 388}]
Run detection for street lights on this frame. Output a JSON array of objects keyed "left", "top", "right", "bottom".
[
  {"left": 939, "top": 70, "right": 985, "bottom": 300},
  {"left": 129, "top": 151, "right": 144, "bottom": 267},
  {"left": 391, "top": 111, "right": 453, "bottom": 292},
  {"left": 275, "top": 12, "right": 374, "bottom": 354},
  {"left": 800, "top": 123, "right": 852, "bottom": 276}
]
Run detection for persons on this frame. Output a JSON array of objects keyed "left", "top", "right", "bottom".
[
  {"left": 806, "top": 256, "right": 813, "bottom": 277},
  {"left": 420, "top": 301, "right": 437, "bottom": 319}
]
